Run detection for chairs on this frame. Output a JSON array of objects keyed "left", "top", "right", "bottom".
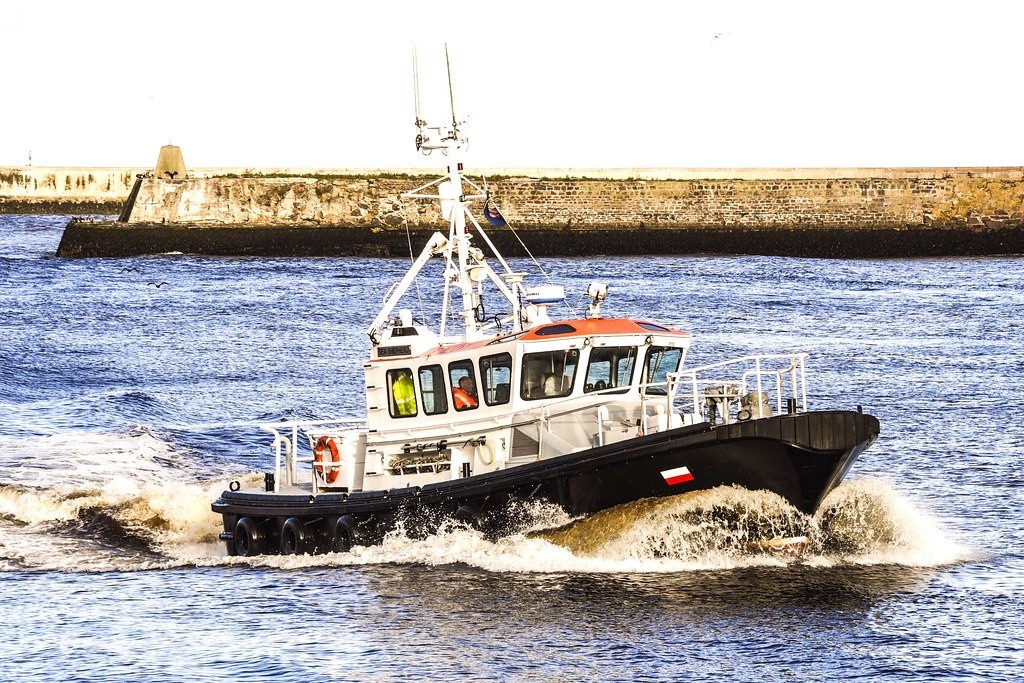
[{"left": 493, "top": 381, "right": 510, "bottom": 402}]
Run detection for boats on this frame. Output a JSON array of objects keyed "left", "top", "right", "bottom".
[{"left": 208, "top": 33, "right": 882, "bottom": 556}]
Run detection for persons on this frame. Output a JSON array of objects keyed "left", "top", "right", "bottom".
[
  {"left": 453, "top": 377, "right": 478, "bottom": 409},
  {"left": 393, "top": 368, "right": 416, "bottom": 414}
]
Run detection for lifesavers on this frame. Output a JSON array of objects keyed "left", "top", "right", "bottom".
[
  {"left": 333, "top": 515, "right": 359, "bottom": 552},
  {"left": 234, "top": 517, "right": 261, "bottom": 557},
  {"left": 280, "top": 517, "right": 306, "bottom": 556},
  {"left": 455, "top": 505, "right": 483, "bottom": 532},
  {"left": 314, "top": 436, "right": 340, "bottom": 483}
]
